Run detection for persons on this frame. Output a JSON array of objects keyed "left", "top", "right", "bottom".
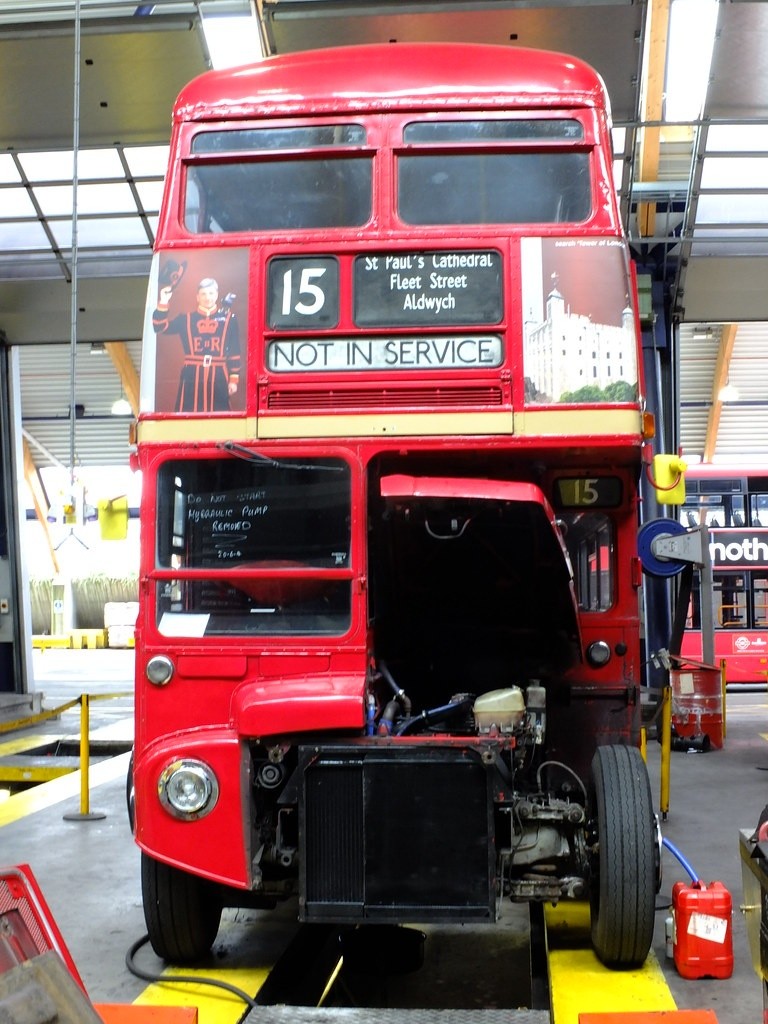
[{"left": 153, "top": 278, "right": 242, "bottom": 411}]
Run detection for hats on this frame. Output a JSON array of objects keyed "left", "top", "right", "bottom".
[{"left": 159, "top": 260, "right": 188, "bottom": 293}]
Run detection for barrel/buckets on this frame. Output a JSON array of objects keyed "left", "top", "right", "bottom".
[
  {"left": 670, "top": 669, "right": 723, "bottom": 751},
  {"left": 672, "top": 881, "right": 734, "bottom": 980}
]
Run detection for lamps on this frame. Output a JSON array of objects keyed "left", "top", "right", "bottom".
[
  {"left": 91, "top": 342, "right": 107, "bottom": 355},
  {"left": 110, "top": 375, "right": 131, "bottom": 415}
]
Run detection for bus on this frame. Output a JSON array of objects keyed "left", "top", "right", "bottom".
[
  {"left": 588, "top": 470, "right": 768, "bottom": 686},
  {"left": 99, "top": 43, "right": 688, "bottom": 968}
]
